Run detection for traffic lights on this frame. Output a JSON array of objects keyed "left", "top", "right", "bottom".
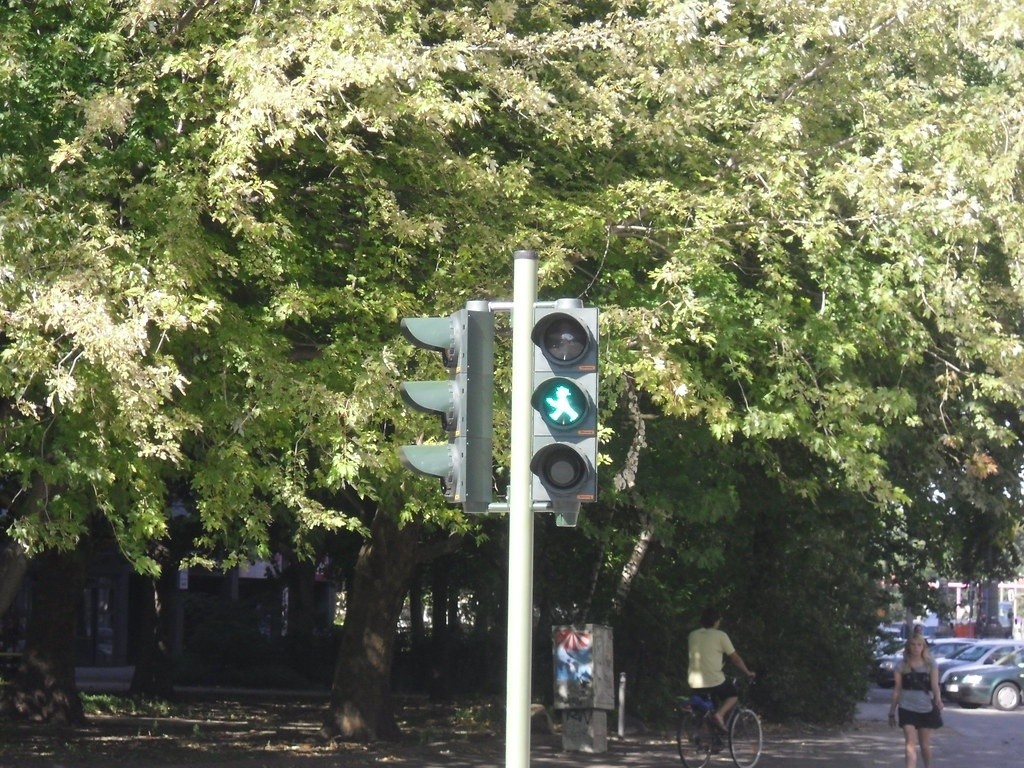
[
  {"left": 532, "top": 297, "right": 607, "bottom": 529},
  {"left": 394, "top": 303, "right": 493, "bottom": 517}
]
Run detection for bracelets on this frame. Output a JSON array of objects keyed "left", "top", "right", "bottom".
[{"left": 887, "top": 713, "right": 895, "bottom": 718}]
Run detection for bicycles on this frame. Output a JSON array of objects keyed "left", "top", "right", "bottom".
[{"left": 668, "top": 676, "right": 765, "bottom": 767}]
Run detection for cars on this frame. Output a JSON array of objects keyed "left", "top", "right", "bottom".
[{"left": 879, "top": 622, "right": 1023, "bottom": 713}]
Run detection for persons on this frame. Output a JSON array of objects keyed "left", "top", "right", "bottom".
[
  {"left": 885, "top": 634, "right": 947, "bottom": 767},
  {"left": 914, "top": 625, "right": 930, "bottom": 648},
  {"left": 683, "top": 607, "right": 756, "bottom": 746}
]
